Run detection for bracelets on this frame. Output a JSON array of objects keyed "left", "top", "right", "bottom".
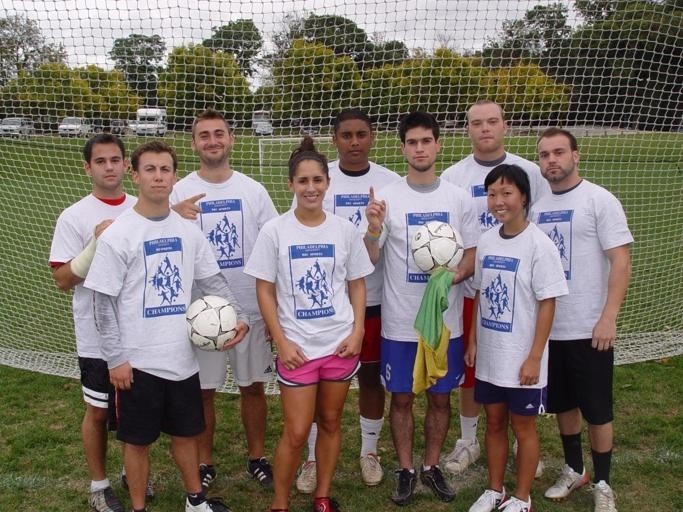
[
  {"left": 71, "top": 234, "right": 98, "bottom": 279},
  {"left": 367, "top": 224, "right": 383, "bottom": 242}
]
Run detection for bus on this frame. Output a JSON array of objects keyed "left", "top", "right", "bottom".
[{"left": 251, "top": 111, "right": 270, "bottom": 130}]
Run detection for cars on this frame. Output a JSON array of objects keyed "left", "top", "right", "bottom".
[
  {"left": 255, "top": 123, "right": 272, "bottom": 135},
  {"left": 300, "top": 127, "right": 317, "bottom": 136}
]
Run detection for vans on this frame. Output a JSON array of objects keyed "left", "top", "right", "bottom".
[
  {"left": 136, "top": 108, "right": 166, "bottom": 136},
  {"left": 0, "top": 118, "right": 33, "bottom": 135},
  {"left": 439, "top": 115, "right": 456, "bottom": 127}
]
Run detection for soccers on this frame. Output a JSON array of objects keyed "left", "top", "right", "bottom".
[
  {"left": 412, "top": 221, "right": 465, "bottom": 270},
  {"left": 186, "top": 296, "right": 238, "bottom": 351}
]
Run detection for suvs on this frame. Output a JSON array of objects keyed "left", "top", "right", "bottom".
[
  {"left": 91, "top": 118, "right": 136, "bottom": 134},
  {"left": 58, "top": 118, "right": 90, "bottom": 136}
]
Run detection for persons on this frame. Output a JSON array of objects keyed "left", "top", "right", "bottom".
[
  {"left": 241, "top": 137, "right": 378, "bottom": 511},
  {"left": 527, "top": 128, "right": 636, "bottom": 512},
  {"left": 85, "top": 141, "right": 252, "bottom": 511},
  {"left": 48, "top": 133, "right": 157, "bottom": 511},
  {"left": 361, "top": 109, "right": 482, "bottom": 507},
  {"left": 463, "top": 164, "right": 570, "bottom": 512},
  {"left": 289, "top": 112, "right": 402, "bottom": 493},
  {"left": 440, "top": 97, "right": 553, "bottom": 480},
  {"left": 164, "top": 108, "right": 280, "bottom": 494}
]
